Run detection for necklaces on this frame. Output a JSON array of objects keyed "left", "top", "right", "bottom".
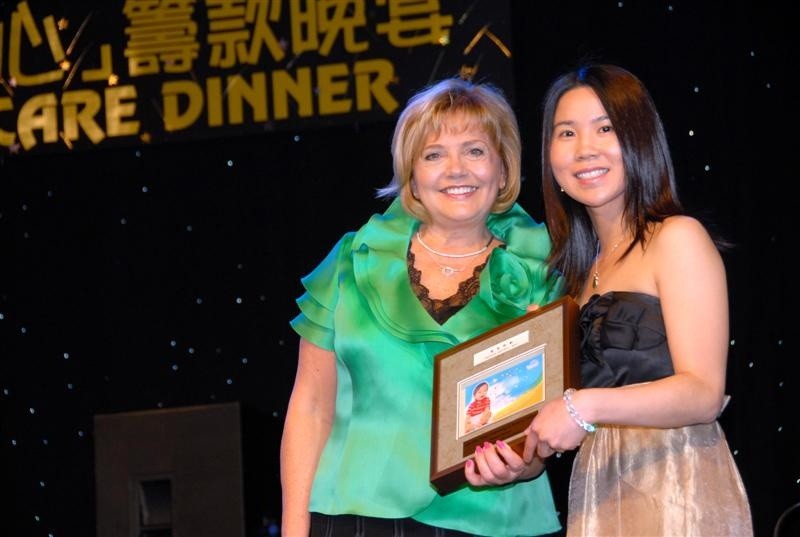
[
  {"left": 592, "top": 230, "right": 630, "bottom": 289},
  {"left": 413, "top": 230, "right": 494, "bottom": 276}
]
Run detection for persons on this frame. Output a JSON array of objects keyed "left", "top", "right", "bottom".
[
  {"left": 281, "top": 76, "right": 582, "bottom": 537},
  {"left": 465, "top": 383, "right": 491, "bottom": 434},
  {"left": 524, "top": 62, "right": 754, "bottom": 537}
]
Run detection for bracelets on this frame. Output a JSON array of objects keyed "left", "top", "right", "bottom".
[{"left": 563, "top": 388, "right": 596, "bottom": 433}]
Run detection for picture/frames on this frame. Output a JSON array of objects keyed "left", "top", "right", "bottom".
[{"left": 429, "top": 294, "right": 582, "bottom": 498}]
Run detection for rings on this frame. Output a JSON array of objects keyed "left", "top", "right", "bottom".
[{"left": 557, "top": 453, "right": 561, "bottom": 457}]
